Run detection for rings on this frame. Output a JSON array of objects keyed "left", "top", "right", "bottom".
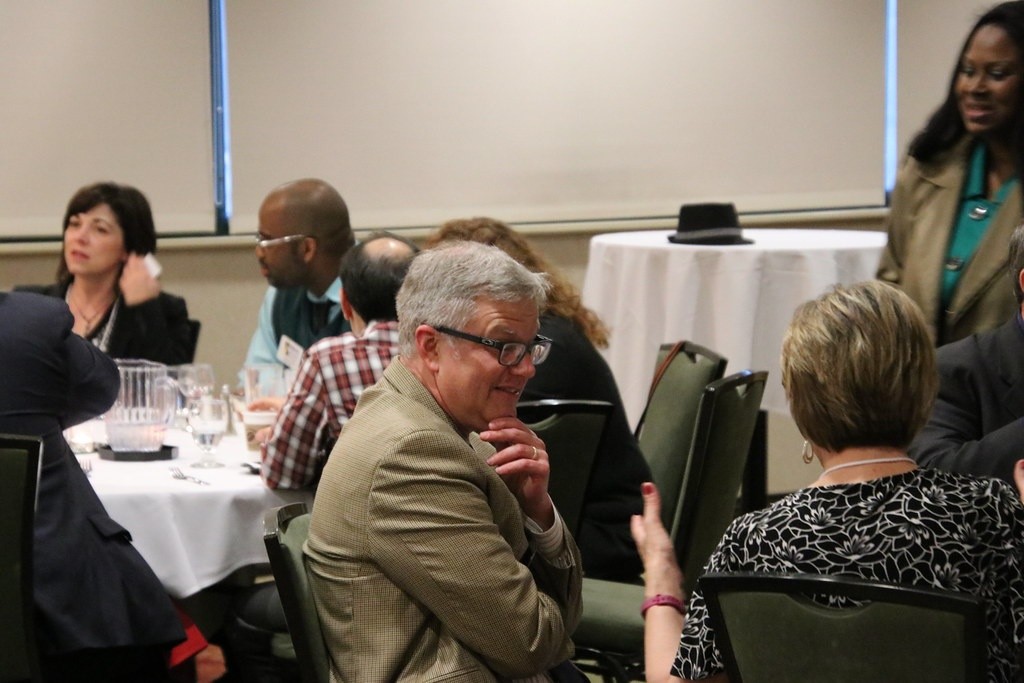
[{"left": 532, "top": 447, "right": 536, "bottom": 459}]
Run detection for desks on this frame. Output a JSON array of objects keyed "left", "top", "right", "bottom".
[
  {"left": 62, "top": 418, "right": 315, "bottom": 638},
  {"left": 584, "top": 227, "right": 889, "bottom": 494}
]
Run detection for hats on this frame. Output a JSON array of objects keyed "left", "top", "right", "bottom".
[{"left": 667, "top": 202, "right": 753, "bottom": 245}]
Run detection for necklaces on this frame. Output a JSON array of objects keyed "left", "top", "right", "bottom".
[{"left": 73, "top": 298, "right": 100, "bottom": 332}]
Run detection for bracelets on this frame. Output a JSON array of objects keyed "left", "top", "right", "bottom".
[{"left": 640, "top": 595, "right": 684, "bottom": 618}]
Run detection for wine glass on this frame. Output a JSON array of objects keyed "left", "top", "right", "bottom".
[{"left": 178, "top": 363, "right": 286, "bottom": 475}]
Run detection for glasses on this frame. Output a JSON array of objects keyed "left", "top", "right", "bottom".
[
  {"left": 254, "top": 232, "right": 308, "bottom": 248},
  {"left": 434, "top": 325, "right": 555, "bottom": 366}
]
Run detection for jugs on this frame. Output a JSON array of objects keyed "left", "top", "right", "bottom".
[{"left": 103, "top": 358, "right": 188, "bottom": 453}]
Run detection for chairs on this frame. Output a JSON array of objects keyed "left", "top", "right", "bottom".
[
  {"left": 0, "top": 433, "right": 329, "bottom": 683},
  {"left": 569, "top": 341, "right": 990, "bottom": 683}
]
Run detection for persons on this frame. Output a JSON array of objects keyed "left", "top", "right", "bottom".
[
  {"left": 873, "top": 1, "right": 1024, "bottom": 345},
  {"left": 630, "top": 280, "right": 1024, "bottom": 682},
  {"left": 220, "top": 233, "right": 421, "bottom": 682},
  {"left": 234, "top": 179, "right": 359, "bottom": 402},
  {"left": 304, "top": 240, "right": 587, "bottom": 683},
  {"left": 910, "top": 225, "right": 1024, "bottom": 487},
  {"left": 13, "top": 182, "right": 189, "bottom": 365},
  {"left": 0, "top": 290, "right": 185, "bottom": 683},
  {"left": 430, "top": 216, "right": 651, "bottom": 585}
]
